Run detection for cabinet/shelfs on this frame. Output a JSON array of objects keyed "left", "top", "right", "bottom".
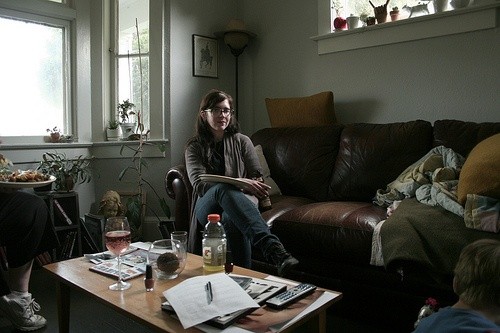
[{"left": 25, "top": 190, "right": 83, "bottom": 273}]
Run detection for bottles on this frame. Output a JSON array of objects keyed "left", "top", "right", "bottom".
[
  {"left": 224, "top": 251, "right": 233, "bottom": 272},
  {"left": 203, "top": 214, "right": 227, "bottom": 271},
  {"left": 144, "top": 265, "right": 154, "bottom": 291},
  {"left": 257, "top": 171, "right": 273, "bottom": 211}
]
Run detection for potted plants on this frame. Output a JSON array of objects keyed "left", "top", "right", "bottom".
[
  {"left": 390, "top": 7, "right": 399, "bottom": 21},
  {"left": 36, "top": 150, "right": 97, "bottom": 192},
  {"left": 47, "top": 127, "right": 61, "bottom": 142},
  {"left": 107, "top": 121, "right": 123, "bottom": 141},
  {"left": 117, "top": 99, "right": 136, "bottom": 137}
]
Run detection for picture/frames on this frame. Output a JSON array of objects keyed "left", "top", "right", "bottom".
[
  {"left": 119, "top": 190, "right": 147, "bottom": 242},
  {"left": 84, "top": 213, "right": 106, "bottom": 251},
  {"left": 192, "top": 34, "right": 218, "bottom": 79}
]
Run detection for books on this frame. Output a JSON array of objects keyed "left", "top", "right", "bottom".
[
  {"left": 58, "top": 231, "right": 77, "bottom": 259},
  {"left": 0, "top": 246, "right": 52, "bottom": 270},
  {"left": 53, "top": 200, "right": 72, "bottom": 225}
]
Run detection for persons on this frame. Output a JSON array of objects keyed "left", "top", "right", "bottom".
[
  {"left": 183, "top": 89, "right": 299, "bottom": 277},
  {"left": 0, "top": 191, "right": 58, "bottom": 331},
  {"left": 410, "top": 239, "right": 500, "bottom": 333},
  {"left": 96, "top": 191, "right": 124, "bottom": 219}
]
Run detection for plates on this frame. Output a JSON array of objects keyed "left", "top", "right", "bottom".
[{"left": 0, "top": 171, "right": 56, "bottom": 187}]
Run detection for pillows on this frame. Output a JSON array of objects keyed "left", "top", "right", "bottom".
[
  {"left": 264, "top": 91, "right": 337, "bottom": 128},
  {"left": 457, "top": 132, "right": 500, "bottom": 204}
]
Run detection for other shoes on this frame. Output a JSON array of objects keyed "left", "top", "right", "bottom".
[{"left": 273, "top": 252, "right": 300, "bottom": 275}]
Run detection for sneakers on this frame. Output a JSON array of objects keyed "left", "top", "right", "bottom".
[{"left": 0, "top": 293, "right": 47, "bottom": 331}]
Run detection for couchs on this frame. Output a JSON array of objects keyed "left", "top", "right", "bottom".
[{"left": 165, "top": 118, "right": 500, "bottom": 328}]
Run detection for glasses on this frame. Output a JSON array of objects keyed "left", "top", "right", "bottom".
[{"left": 204, "top": 107, "right": 235, "bottom": 117}]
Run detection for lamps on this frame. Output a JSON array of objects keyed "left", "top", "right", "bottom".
[{"left": 224, "top": 32, "right": 249, "bottom": 131}]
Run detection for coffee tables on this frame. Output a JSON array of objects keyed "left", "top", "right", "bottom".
[{"left": 42, "top": 241, "right": 343, "bottom": 333}]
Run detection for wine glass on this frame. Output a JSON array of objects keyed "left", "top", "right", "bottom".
[{"left": 104, "top": 217, "right": 131, "bottom": 290}]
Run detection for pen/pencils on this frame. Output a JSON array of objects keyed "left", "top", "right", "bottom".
[{"left": 206, "top": 281, "right": 213, "bottom": 301}]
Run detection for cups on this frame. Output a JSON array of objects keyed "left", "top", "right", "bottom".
[{"left": 171, "top": 231, "right": 187, "bottom": 259}]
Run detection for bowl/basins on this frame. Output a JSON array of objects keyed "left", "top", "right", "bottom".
[{"left": 148, "top": 239, "right": 186, "bottom": 280}]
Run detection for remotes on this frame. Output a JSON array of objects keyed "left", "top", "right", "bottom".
[{"left": 265, "top": 284, "right": 317, "bottom": 311}]
